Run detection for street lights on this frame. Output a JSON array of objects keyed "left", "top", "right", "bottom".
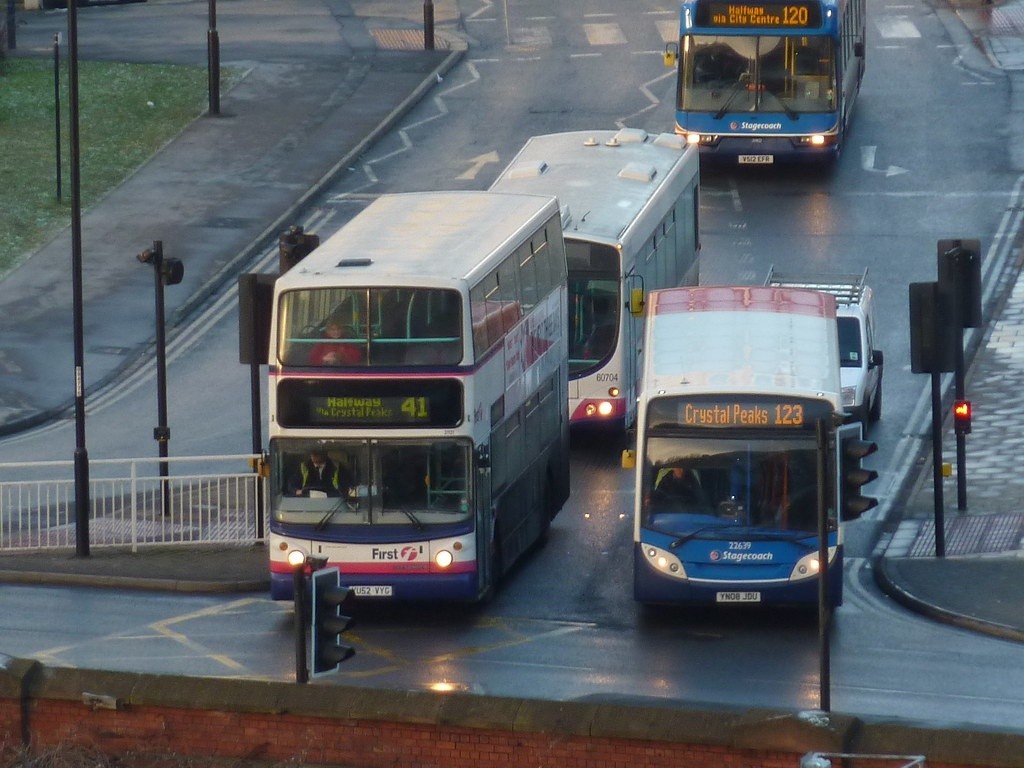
[{"left": 138, "top": 244, "right": 186, "bottom": 515}]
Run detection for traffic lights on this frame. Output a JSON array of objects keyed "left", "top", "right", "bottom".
[
  {"left": 307, "top": 566, "right": 356, "bottom": 680},
  {"left": 953, "top": 401, "right": 970, "bottom": 437},
  {"left": 834, "top": 423, "right": 879, "bottom": 524}
]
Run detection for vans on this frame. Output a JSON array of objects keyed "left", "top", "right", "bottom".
[{"left": 762, "top": 284, "right": 883, "bottom": 437}]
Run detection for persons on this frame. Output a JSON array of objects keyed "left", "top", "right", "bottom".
[
  {"left": 293, "top": 447, "right": 352, "bottom": 497},
  {"left": 654, "top": 467, "right": 702, "bottom": 505},
  {"left": 308, "top": 321, "right": 362, "bottom": 365}
]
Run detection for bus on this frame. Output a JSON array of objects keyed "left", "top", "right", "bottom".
[
  {"left": 264, "top": 190, "right": 569, "bottom": 608},
  {"left": 621, "top": 286, "right": 844, "bottom": 622},
  {"left": 488, "top": 128, "right": 699, "bottom": 431},
  {"left": 664, "top": 1, "right": 867, "bottom": 174}
]
